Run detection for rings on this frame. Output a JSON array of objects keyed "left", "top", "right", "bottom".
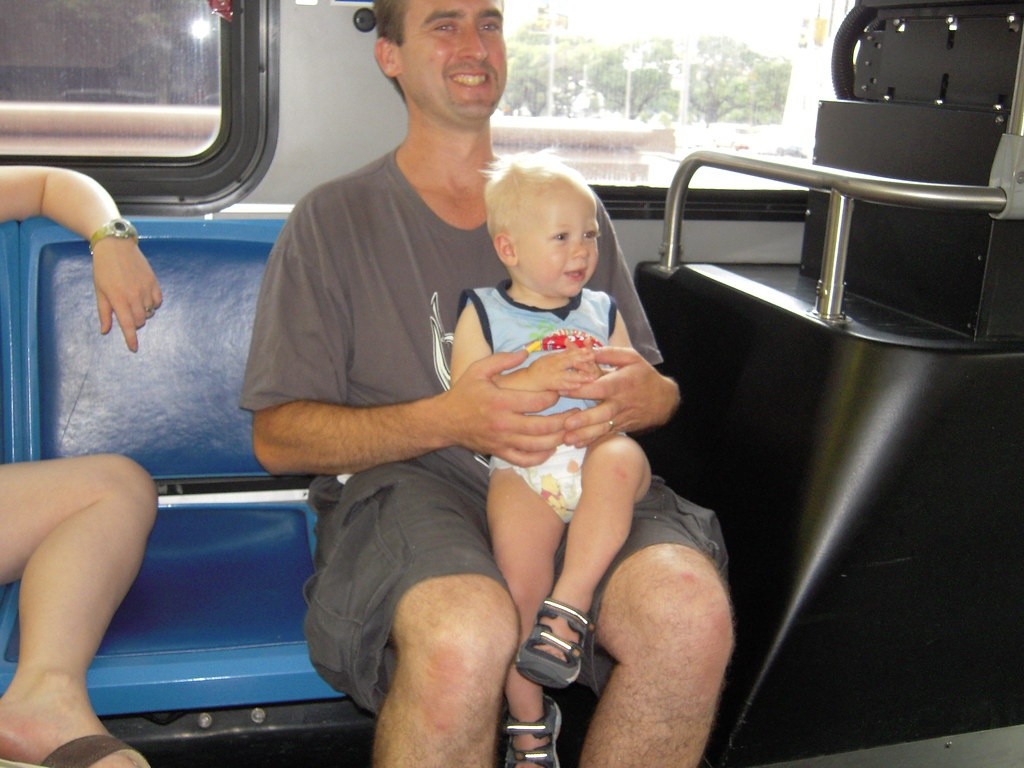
[
  {"left": 608, "top": 420, "right": 614, "bottom": 431},
  {"left": 146, "top": 308, "right": 153, "bottom": 312}
]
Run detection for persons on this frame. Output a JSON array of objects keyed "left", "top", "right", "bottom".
[
  {"left": 0, "top": 166, "right": 164, "bottom": 768},
  {"left": 238, "top": 0, "right": 734, "bottom": 767},
  {"left": 452, "top": 154, "right": 651, "bottom": 768}
]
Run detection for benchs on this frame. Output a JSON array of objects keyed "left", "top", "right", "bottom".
[{"left": 0, "top": 213, "right": 348, "bottom": 714}]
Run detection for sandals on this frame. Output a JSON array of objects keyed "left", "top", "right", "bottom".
[
  {"left": 503, "top": 695, "right": 563, "bottom": 768},
  {"left": 515, "top": 597, "right": 590, "bottom": 688}
]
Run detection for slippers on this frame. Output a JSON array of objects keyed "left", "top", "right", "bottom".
[{"left": 0, "top": 734, "right": 149, "bottom": 767}]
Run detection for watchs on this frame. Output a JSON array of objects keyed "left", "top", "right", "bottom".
[{"left": 88, "top": 218, "right": 140, "bottom": 253}]
